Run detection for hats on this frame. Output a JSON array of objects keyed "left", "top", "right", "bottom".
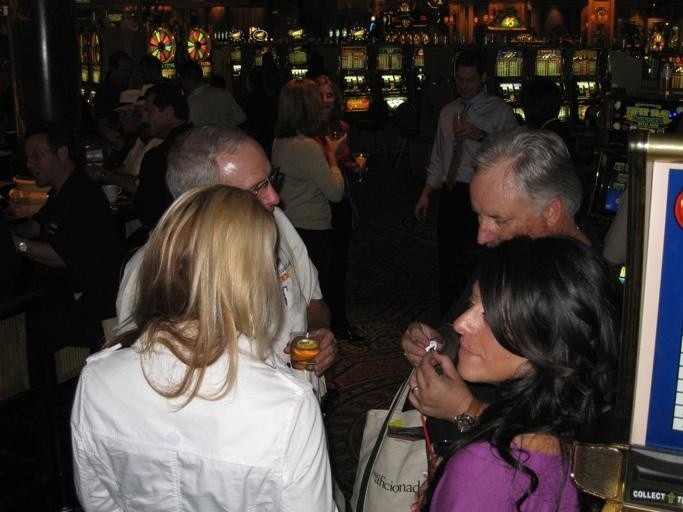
[
  {"left": 113, "top": 88, "right": 135, "bottom": 113},
  {"left": 134, "top": 83, "right": 152, "bottom": 105}
]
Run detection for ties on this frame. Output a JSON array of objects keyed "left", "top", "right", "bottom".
[{"left": 445, "top": 104, "right": 471, "bottom": 190}]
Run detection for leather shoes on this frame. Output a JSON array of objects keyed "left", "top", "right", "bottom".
[{"left": 335, "top": 327, "right": 365, "bottom": 340}]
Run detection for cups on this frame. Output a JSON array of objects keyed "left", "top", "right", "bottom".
[
  {"left": 328, "top": 127, "right": 345, "bottom": 153},
  {"left": 289, "top": 336, "right": 321, "bottom": 370},
  {"left": 104, "top": 185, "right": 121, "bottom": 203}
]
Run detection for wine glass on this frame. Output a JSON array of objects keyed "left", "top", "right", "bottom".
[
  {"left": 352, "top": 153, "right": 367, "bottom": 182},
  {"left": 453, "top": 112, "right": 463, "bottom": 156}
]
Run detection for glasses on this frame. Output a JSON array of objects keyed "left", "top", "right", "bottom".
[
  {"left": 22, "top": 144, "right": 58, "bottom": 162},
  {"left": 251, "top": 165, "right": 282, "bottom": 194}
]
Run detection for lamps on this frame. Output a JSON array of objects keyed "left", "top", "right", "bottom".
[{"left": 489, "top": 9, "right": 527, "bottom": 32}]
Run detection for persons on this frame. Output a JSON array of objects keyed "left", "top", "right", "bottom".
[
  {"left": 116, "top": 123, "right": 339, "bottom": 404},
  {"left": 69, "top": 184, "right": 345, "bottom": 512},
  {"left": 401, "top": 125, "right": 624, "bottom": 434},
  {"left": 411, "top": 233, "right": 623, "bottom": 512},
  {"left": 0, "top": 49, "right": 364, "bottom": 339},
  {"left": 414, "top": 48, "right": 562, "bottom": 322}
]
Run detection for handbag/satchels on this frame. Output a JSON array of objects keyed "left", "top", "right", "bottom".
[{"left": 349, "top": 407, "right": 431, "bottom": 511}]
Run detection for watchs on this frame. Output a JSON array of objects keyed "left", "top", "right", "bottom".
[{"left": 455, "top": 399, "right": 483, "bottom": 433}]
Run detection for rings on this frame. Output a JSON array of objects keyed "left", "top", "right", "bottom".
[{"left": 412, "top": 386, "right": 420, "bottom": 394}]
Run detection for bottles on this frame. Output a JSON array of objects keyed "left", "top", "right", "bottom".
[{"left": 659, "top": 62, "right": 673, "bottom": 98}]
